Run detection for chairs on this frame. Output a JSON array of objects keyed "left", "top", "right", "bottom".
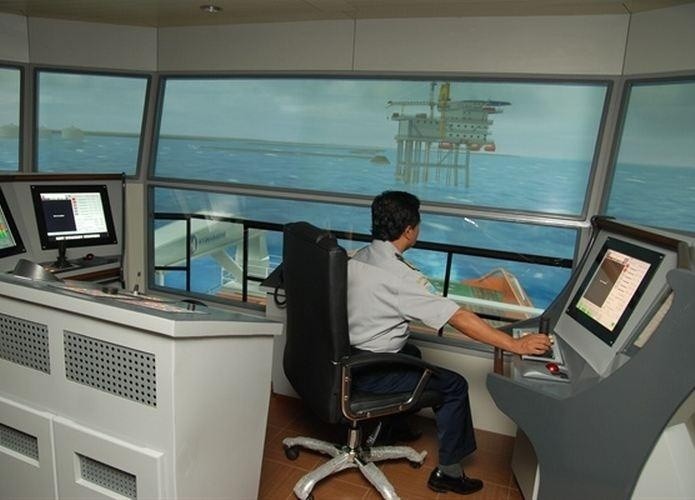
[{"left": 278, "top": 220, "right": 441, "bottom": 499}]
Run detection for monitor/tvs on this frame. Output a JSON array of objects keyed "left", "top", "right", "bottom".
[
  {"left": 0, "top": 186, "right": 27, "bottom": 259},
  {"left": 600, "top": 283, "right": 675, "bottom": 383},
  {"left": 30, "top": 184, "right": 118, "bottom": 273},
  {"left": 553, "top": 230, "right": 678, "bottom": 378}
]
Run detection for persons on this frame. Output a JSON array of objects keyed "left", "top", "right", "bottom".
[{"left": 346, "top": 189, "right": 554, "bottom": 495}]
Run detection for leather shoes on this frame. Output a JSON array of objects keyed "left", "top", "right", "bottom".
[
  {"left": 379, "top": 428, "right": 423, "bottom": 444},
  {"left": 427, "top": 466, "right": 483, "bottom": 495}
]
